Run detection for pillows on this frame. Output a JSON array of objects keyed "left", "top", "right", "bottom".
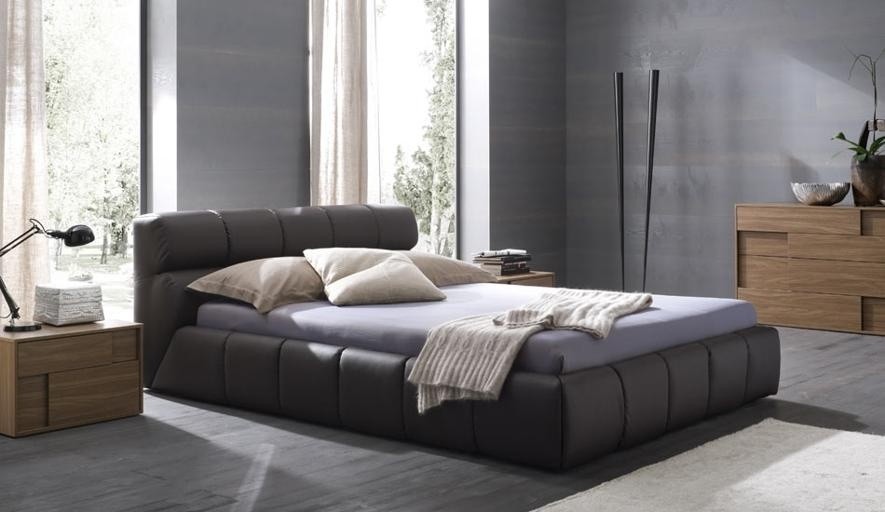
[
  {"left": 186, "top": 257, "right": 325, "bottom": 315},
  {"left": 397, "top": 250, "right": 500, "bottom": 287},
  {"left": 303, "top": 248, "right": 447, "bottom": 305}
]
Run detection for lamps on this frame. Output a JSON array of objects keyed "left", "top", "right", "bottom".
[{"left": 0, "top": 217, "right": 95, "bottom": 331}]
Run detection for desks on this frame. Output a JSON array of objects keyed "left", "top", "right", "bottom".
[{"left": 734, "top": 202, "right": 885, "bottom": 337}]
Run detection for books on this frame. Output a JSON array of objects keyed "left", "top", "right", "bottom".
[{"left": 472, "top": 248, "right": 532, "bottom": 276}]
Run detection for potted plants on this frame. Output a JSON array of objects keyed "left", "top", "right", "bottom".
[{"left": 829, "top": 43, "right": 885, "bottom": 207}]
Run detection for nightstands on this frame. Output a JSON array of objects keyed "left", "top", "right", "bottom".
[
  {"left": 495, "top": 269, "right": 555, "bottom": 287},
  {"left": 0, "top": 315, "right": 144, "bottom": 438}
]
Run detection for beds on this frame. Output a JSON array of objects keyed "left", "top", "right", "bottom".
[{"left": 134, "top": 204, "right": 781, "bottom": 476}]
